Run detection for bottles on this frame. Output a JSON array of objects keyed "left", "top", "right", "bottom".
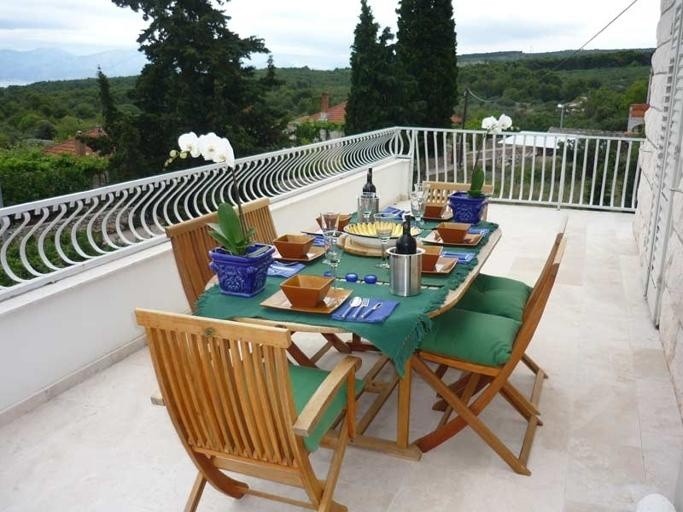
[
  {"left": 358, "top": 167, "right": 378, "bottom": 222},
  {"left": 395, "top": 215, "right": 416, "bottom": 253}
]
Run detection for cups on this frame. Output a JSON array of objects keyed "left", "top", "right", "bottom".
[
  {"left": 345, "top": 273, "right": 358, "bottom": 282},
  {"left": 364, "top": 275, "right": 376, "bottom": 283}
]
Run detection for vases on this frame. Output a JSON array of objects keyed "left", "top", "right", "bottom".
[
  {"left": 207, "top": 242, "right": 272, "bottom": 299},
  {"left": 447, "top": 190, "right": 490, "bottom": 222}
]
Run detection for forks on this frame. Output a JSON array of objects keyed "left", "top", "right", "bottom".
[{"left": 351, "top": 298, "right": 369, "bottom": 318}]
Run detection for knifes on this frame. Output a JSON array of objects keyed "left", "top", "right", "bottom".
[{"left": 360, "top": 303, "right": 382, "bottom": 319}]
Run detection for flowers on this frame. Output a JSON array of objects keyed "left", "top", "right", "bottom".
[
  {"left": 160, "top": 130, "right": 258, "bottom": 257},
  {"left": 463, "top": 113, "right": 513, "bottom": 197}
]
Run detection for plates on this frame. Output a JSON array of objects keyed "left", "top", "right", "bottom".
[
  {"left": 259, "top": 286, "right": 352, "bottom": 313},
  {"left": 266, "top": 243, "right": 326, "bottom": 263},
  {"left": 420, "top": 230, "right": 483, "bottom": 247},
  {"left": 419, "top": 212, "right": 454, "bottom": 221},
  {"left": 420, "top": 257, "right": 458, "bottom": 276}
]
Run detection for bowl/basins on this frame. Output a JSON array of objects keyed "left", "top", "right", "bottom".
[
  {"left": 272, "top": 232, "right": 315, "bottom": 257},
  {"left": 279, "top": 274, "right": 333, "bottom": 307},
  {"left": 315, "top": 214, "right": 351, "bottom": 231},
  {"left": 341, "top": 223, "right": 422, "bottom": 250},
  {"left": 436, "top": 222, "right": 472, "bottom": 242},
  {"left": 424, "top": 202, "right": 447, "bottom": 217},
  {"left": 419, "top": 245, "right": 444, "bottom": 271}
]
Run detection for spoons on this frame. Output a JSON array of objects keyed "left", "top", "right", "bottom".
[{"left": 340, "top": 296, "right": 361, "bottom": 320}]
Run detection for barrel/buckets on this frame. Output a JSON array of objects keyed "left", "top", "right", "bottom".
[
  {"left": 357, "top": 196, "right": 380, "bottom": 224},
  {"left": 385, "top": 247, "right": 427, "bottom": 299}
]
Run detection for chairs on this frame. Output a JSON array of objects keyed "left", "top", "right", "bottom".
[
  {"left": 167, "top": 213, "right": 351, "bottom": 370},
  {"left": 133, "top": 307, "right": 372, "bottom": 512},
  {"left": 409, "top": 234, "right": 572, "bottom": 478},
  {"left": 430, "top": 215, "right": 572, "bottom": 414},
  {"left": 229, "top": 196, "right": 279, "bottom": 244},
  {"left": 420, "top": 179, "right": 495, "bottom": 223}
]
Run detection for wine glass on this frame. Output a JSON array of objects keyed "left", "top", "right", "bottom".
[
  {"left": 372, "top": 213, "right": 392, "bottom": 269},
  {"left": 318, "top": 210, "right": 346, "bottom": 284},
  {"left": 411, "top": 182, "right": 430, "bottom": 236}
]
